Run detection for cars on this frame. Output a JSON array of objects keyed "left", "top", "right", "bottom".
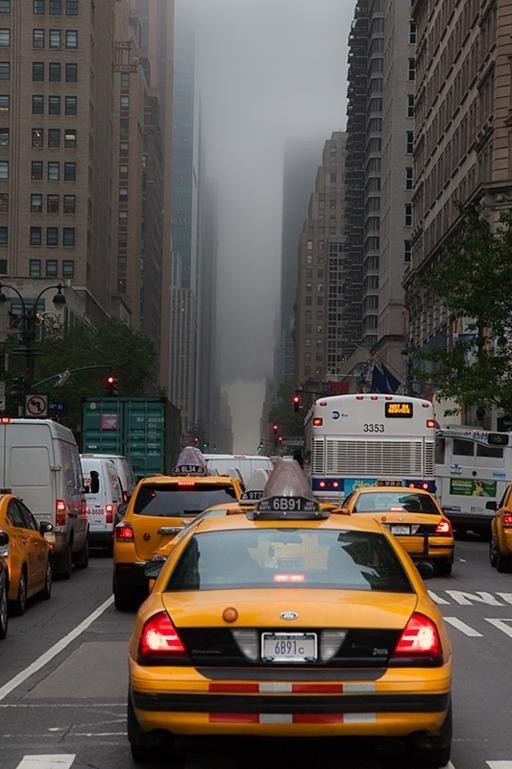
[
  {"left": 1, "top": 488, "right": 55, "bottom": 635},
  {"left": 488, "top": 483, "right": 512, "bottom": 574}
]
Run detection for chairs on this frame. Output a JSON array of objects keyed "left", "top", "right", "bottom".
[
  {"left": 401, "top": 500, "right": 421, "bottom": 513},
  {"left": 326, "top": 543, "right": 373, "bottom": 587},
  {"left": 210, "top": 541, "right": 258, "bottom": 584}
]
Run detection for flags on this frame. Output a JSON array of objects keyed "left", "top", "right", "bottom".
[
  {"left": 370, "top": 365, "right": 390, "bottom": 393},
  {"left": 380, "top": 362, "right": 402, "bottom": 393},
  {"left": 329, "top": 381, "right": 350, "bottom": 394}
]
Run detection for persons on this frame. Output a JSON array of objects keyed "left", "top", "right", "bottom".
[{"left": 472, "top": 481, "right": 484, "bottom": 496}]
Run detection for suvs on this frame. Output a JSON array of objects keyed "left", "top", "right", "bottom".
[{"left": 112, "top": 447, "right": 253, "bottom": 613}]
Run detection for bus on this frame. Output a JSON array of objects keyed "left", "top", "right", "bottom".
[{"left": 301, "top": 392, "right": 437, "bottom": 505}]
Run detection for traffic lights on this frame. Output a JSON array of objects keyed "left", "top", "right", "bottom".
[
  {"left": 109, "top": 375, "right": 118, "bottom": 396},
  {"left": 273, "top": 422, "right": 278, "bottom": 435},
  {"left": 293, "top": 394, "right": 301, "bottom": 414},
  {"left": 195, "top": 436, "right": 200, "bottom": 449},
  {"left": 275, "top": 437, "right": 283, "bottom": 448},
  {"left": 256, "top": 442, "right": 263, "bottom": 453},
  {"left": 202, "top": 438, "right": 208, "bottom": 452}
]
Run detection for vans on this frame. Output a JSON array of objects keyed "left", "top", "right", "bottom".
[
  {"left": 76, "top": 450, "right": 136, "bottom": 553},
  {"left": 2, "top": 418, "right": 89, "bottom": 581}
]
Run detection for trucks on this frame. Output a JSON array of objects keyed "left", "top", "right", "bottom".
[
  {"left": 82, "top": 394, "right": 180, "bottom": 484},
  {"left": 434, "top": 418, "right": 512, "bottom": 540}
]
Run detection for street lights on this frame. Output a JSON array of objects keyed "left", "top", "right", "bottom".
[{"left": 0, "top": 283, "right": 69, "bottom": 417}]
